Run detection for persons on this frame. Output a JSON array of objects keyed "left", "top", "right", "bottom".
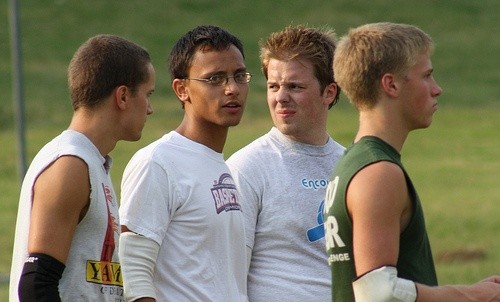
[
  {"left": 322, "top": 21, "right": 500, "bottom": 302},
  {"left": 10, "top": 34, "right": 155, "bottom": 302},
  {"left": 117, "top": 25, "right": 250, "bottom": 302},
  {"left": 224, "top": 22, "right": 346, "bottom": 301}
]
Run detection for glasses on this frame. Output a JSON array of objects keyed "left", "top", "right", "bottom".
[{"left": 179, "top": 72, "right": 255, "bottom": 86}]
